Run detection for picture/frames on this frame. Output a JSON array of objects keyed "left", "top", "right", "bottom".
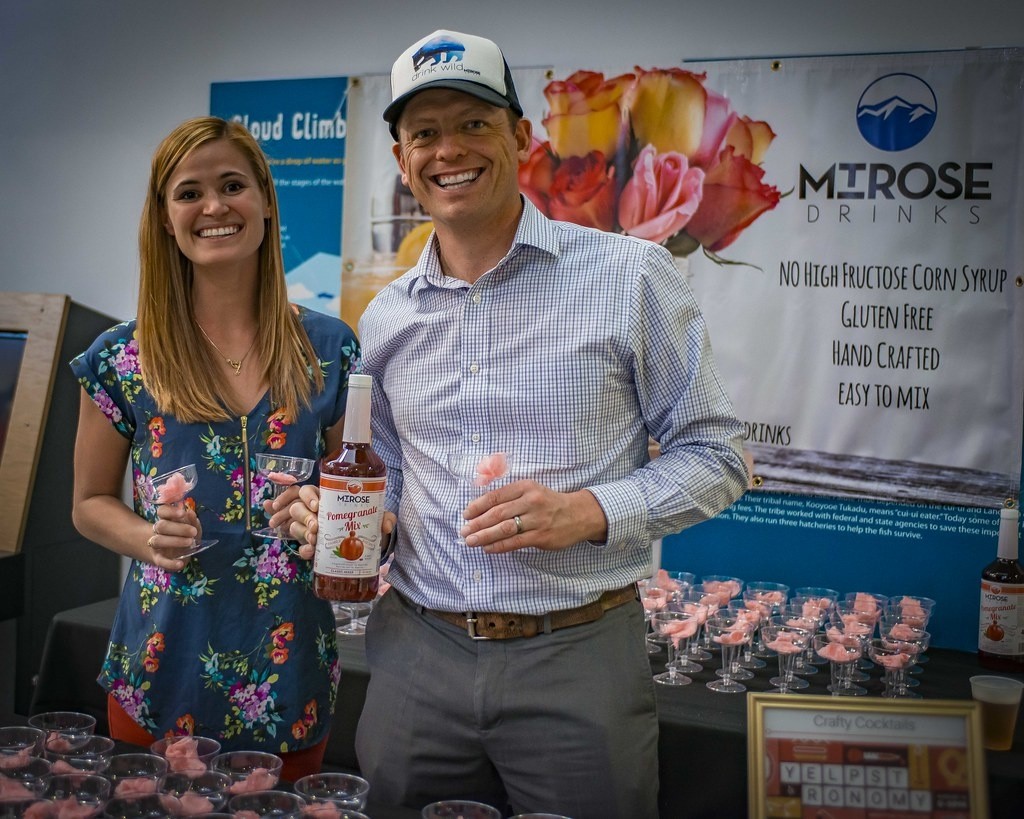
[
  {"left": 0, "top": 288, "right": 69, "bottom": 558},
  {"left": 746, "top": 691, "right": 988, "bottom": 819}
]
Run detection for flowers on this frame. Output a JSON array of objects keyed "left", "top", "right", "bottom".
[{"left": 514, "top": 66, "right": 790, "bottom": 272}]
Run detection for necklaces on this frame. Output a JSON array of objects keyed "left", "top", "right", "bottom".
[{"left": 194, "top": 318, "right": 260, "bottom": 376}]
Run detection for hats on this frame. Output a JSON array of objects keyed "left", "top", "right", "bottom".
[{"left": 382, "top": 30, "right": 523, "bottom": 142}]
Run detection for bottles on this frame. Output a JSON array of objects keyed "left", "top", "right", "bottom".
[
  {"left": 314, "top": 373, "right": 388, "bottom": 602},
  {"left": 978, "top": 507, "right": 1024, "bottom": 679}
]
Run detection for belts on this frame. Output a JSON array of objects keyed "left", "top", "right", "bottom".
[{"left": 422, "top": 582, "right": 637, "bottom": 641}]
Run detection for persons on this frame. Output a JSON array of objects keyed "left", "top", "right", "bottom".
[
  {"left": 289, "top": 29, "right": 752, "bottom": 819},
  {"left": 69, "top": 118, "right": 361, "bottom": 785}
]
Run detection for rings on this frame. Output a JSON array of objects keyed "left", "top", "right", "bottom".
[
  {"left": 148, "top": 536, "right": 160, "bottom": 550},
  {"left": 513, "top": 516, "right": 524, "bottom": 534},
  {"left": 152, "top": 522, "right": 157, "bottom": 534}
]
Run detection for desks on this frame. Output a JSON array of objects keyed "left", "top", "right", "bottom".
[{"left": 35, "top": 598, "right": 1024, "bottom": 819}]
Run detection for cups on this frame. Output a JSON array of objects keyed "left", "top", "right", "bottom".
[{"left": 970, "top": 676, "right": 1024, "bottom": 751}]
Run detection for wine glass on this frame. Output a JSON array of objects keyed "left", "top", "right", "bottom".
[
  {"left": 251, "top": 453, "right": 315, "bottom": 540},
  {"left": 331, "top": 602, "right": 370, "bottom": 636},
  {"left": 448, "top": 451, "right": 510, "bottom": 546},
  {"left": 0, "top": 710, "right": 576, "bottom": 819},
  {"left": 637, "top": 571, "right": 936, "bottom": 697},
  {"left": 136, "top": 462, "right": 219, "bottom": 559}
]
